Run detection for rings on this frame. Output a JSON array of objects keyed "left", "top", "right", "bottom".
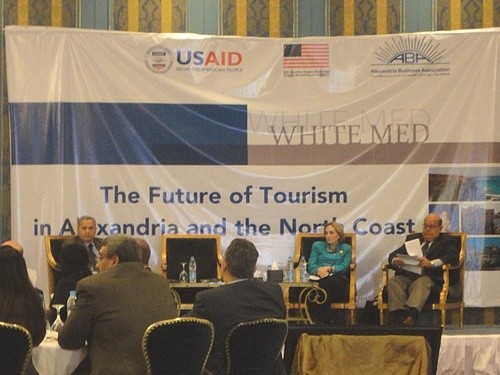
[{"left": 399, "top": 266, "right": 401, "bottom": 268}]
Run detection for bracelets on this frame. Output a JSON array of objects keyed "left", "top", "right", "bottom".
[
  {"left": 331, "top": 266, "right": 334, "bottom": 273},
  {"left": 430, "top": 261, "right": 434, "bottom": 267}
]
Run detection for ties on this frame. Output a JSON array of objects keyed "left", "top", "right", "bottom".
[
  {"left": 421, "top": 241, "right": 430, "bottom": 256},
  {"left": 88, "top": 244, "right": 97, "bottom": 270}
]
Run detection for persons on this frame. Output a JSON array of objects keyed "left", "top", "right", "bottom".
[
  {"left": 307, "top": 222, "right": 352, "bottom": 327},
  {"left": 58, "top": 234, "right": 178, "bottom": 375},
  {"left": 0, "top": 240, "right": 23, "bottom": 256},
  {"left": 135, "top": 237, "right": 152, "bottom": 271},
  {"left": 0, "top": 245, "right": 46, "bottom": 375},
  {"left": 190, "top": 238, "right": 287, "bottom": 375},
  {"left": 388, "top": 214, "right": 458, "bottom": 325},
  {"left": 49, "top": 216, "right": 103, "bottom": 331}
]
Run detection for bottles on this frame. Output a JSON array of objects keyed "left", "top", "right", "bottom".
[
  {"left": 298, "top": 256, "right": 307, "bottom": 282},
  {"left": 66, "top": 291, "right": 77, "bottom": 320},
  {"left": 287, "top": 256, "right": 294, "bottom": 282},
  {"left": 188, "top": 256, "right": 197, "bottom": 283}
]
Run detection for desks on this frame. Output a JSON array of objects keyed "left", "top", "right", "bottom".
[
  {"left": 171, "top": 283, "right": 328, "bottom": 325},
  {"left": 435, "top": 334, "right": 500, "bottom": 375},
  {"left": 31, "top": 334, "right": 88, "bottom": 375}
]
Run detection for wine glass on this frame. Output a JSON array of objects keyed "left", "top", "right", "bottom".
[
  {"left": 179, "top": 262, "right": 189, "bottom": 284},
  {"left": 301, "top": 261, "right": 309, "bottom": 283},
  {"left": 50, "top": 303, "right": 66, "bottom": 341}
]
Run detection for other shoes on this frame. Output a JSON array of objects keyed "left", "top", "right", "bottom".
[
  {"left": 403, "top": 316, "right": 415, "bottom": 326},
  {"left": 392, "top": 317, "right": 402, "bottom": 327}
]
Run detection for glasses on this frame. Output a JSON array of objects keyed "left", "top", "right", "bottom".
[{"left": 422, "top": 223, "right": 441, "bottom": 230}]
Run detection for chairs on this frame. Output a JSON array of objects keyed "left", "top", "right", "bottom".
[
  {"left": 285, "top": 233, "right": 358, "bottom": 326},
  {"left": 0, "top": 321, "right": 33, "bottom": 375},
  {"left": 141, "top": 316, "right": 215, "bottom": 375},
  {"left": 161, "top": 235, "right": 224, "bottom": 284},
  {"left": 377, "top": 233, "right": 467, "bottom": 327},
  {"left": 45, "top": 235, "right": 70, "bottom": 303},
  {"left": 223, "top": 318, "right": 288, "bottom": 375}
]
